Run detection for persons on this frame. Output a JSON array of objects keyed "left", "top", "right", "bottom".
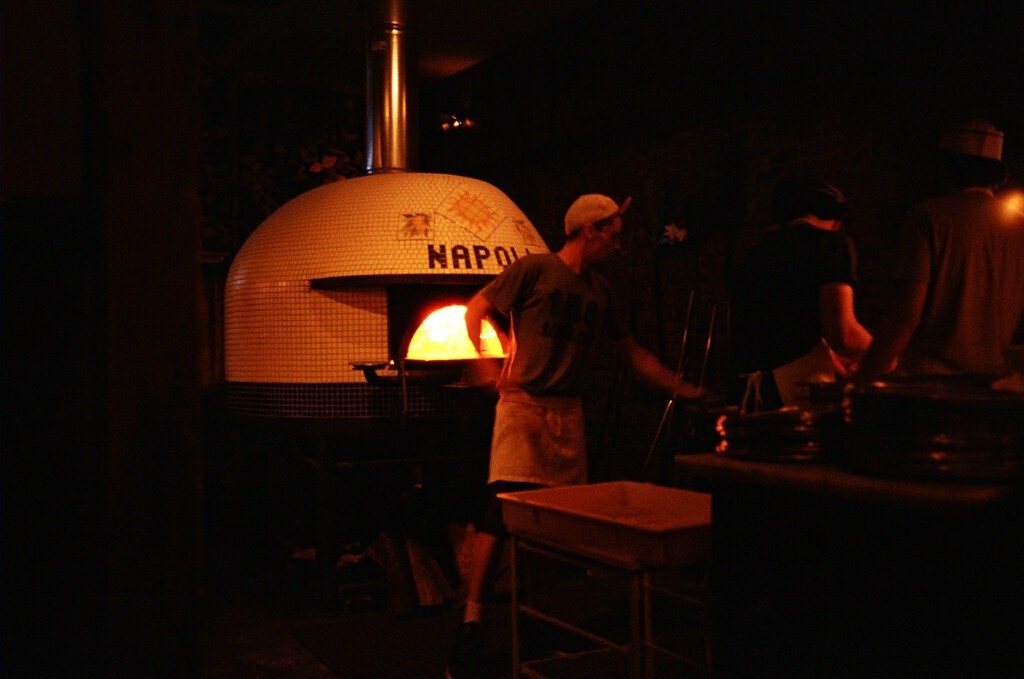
[
  {"left": 449, "top": 193, "right": 724, "bottom": 639},
  {"left": 732, "top": 190, "right": 877, "bottom": 414},
  {"left": 849, "top": 120, "right": 1024, "bottom": 377}
]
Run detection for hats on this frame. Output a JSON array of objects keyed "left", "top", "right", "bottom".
[
  {"left": 564, "top": 194, "right": 624, "bottom": 235},
  {"left": 962, "top": 122, "right": 1004, "bottom": 160}
]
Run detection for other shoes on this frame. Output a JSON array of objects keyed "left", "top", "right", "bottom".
[
  {"left": 395, "top": 600, "right": 450, "bottom": 619},
  {"left": 446, "top": 622, "right": 482, "bottom": 679}
]
